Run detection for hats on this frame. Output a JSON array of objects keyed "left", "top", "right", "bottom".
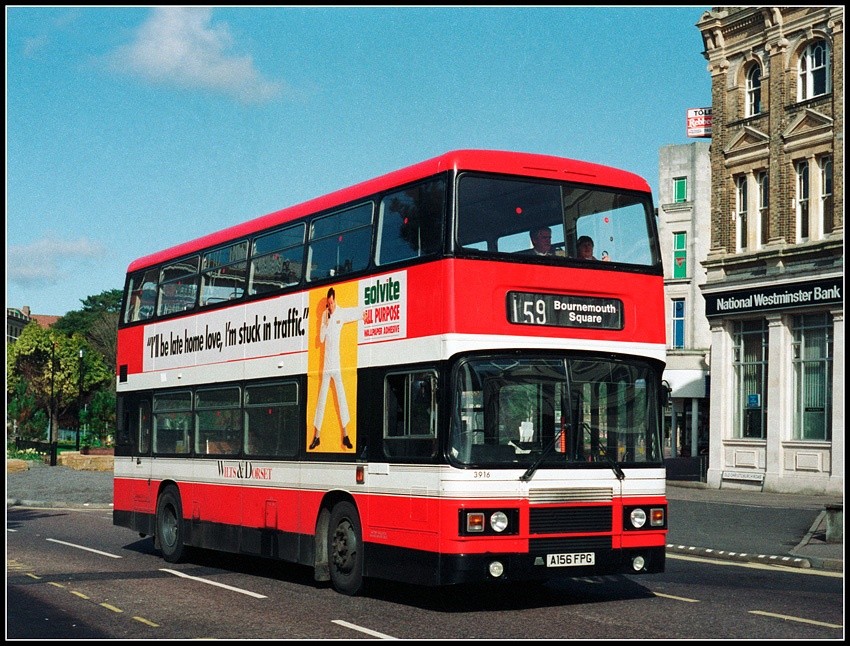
[{"left": 577, "top": 236, "right": 594, "bottom": 246}]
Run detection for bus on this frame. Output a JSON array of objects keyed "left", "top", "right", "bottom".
[{"left": 112, "top": 147, "right": 670, "bottom": 597}]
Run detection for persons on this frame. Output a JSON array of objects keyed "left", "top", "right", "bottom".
[
  {"left": 309, "top": 287, "right": 360, "bottom": 449},
  {"left": 413, "top": 406, "right": 434, "bottom": 433},
  {"left": 523, "top": 226, "right": 565, "bottom": 256},
  {"left": 579, "top": 236, "right": 595, "bottom": 260}
]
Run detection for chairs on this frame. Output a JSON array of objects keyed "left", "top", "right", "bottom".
[{"left": 205, "top": 433, "right": 234, "bottom": 454}]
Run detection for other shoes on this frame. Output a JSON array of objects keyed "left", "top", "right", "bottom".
[
  {"left": 343, "top": 436, "right": 353, "bottom": 449},
  {"left": 309, "top": 437, "right": 320, "bottom": 449}
]
